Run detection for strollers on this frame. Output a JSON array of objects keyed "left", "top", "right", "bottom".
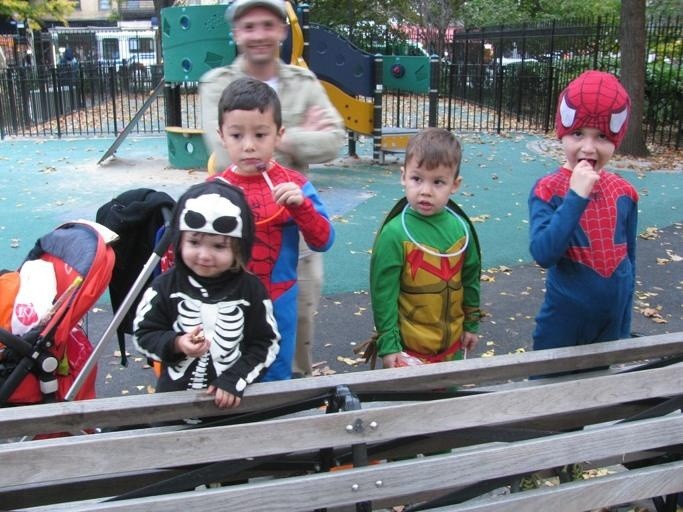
[{"left": 0, "top": 190, "right": 179, "bottom": 442}]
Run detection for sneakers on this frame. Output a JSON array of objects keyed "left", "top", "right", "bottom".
[{"left": 512, "top": 464, "right": 584, "bottom": 491}]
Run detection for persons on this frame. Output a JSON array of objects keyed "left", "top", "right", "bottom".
[
  {"left": 158, "top": 76, "right": 335, "bottom": 383},
  {"left": 194, "top": 0, "right": 349, "bottom": 382},
  {"left": 127, "top": 178, "right": 281, "bottom": 493},
  {"left": 366, "top": 127, "right": 483, "bottom": 462},
  {"left": 522, "top": 65, "right": 642, "bottom": 434}
]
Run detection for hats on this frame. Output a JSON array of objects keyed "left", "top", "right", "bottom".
[
  {"left": 557, "top": 71, "right": 631, "bottom": 149},
  {"left": 226, "top": 0, "right": 288, "bottom": 21},
  {"left": 179, "top": 183, "right": 249, "bottom": 240}
]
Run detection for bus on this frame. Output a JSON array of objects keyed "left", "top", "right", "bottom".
[
  {"left": 361, "top": 40, "right": 432, "bottom": 60},
  {"left": 47, "top": 14, "right": 161, "bottom": 91}
]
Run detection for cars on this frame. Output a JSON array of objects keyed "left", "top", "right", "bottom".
[{"left": 485, "top": 48, "right": 566, "bottom": 89}]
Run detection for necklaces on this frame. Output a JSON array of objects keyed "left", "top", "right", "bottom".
[{"left": 399, "top": 201, "right": 473, "bottom": 260}]
